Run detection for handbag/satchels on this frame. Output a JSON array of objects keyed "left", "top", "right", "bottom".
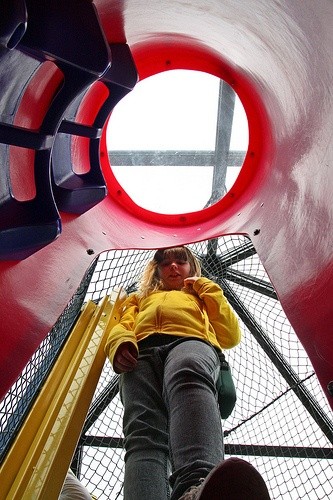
[{"left": 215, "top": 361, "right": 236, "bottom": 419}]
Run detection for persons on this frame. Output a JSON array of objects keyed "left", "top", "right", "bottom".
[
  {"left": 59, "top": 466, "right": 95, "bottom": 500},
  {"left": 101, "top": 245, "right": 271, "bottom": 500}
]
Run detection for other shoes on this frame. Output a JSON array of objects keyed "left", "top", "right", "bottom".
[{"left": 187, "top": 457, "right": 270, "bottom": 500}]
